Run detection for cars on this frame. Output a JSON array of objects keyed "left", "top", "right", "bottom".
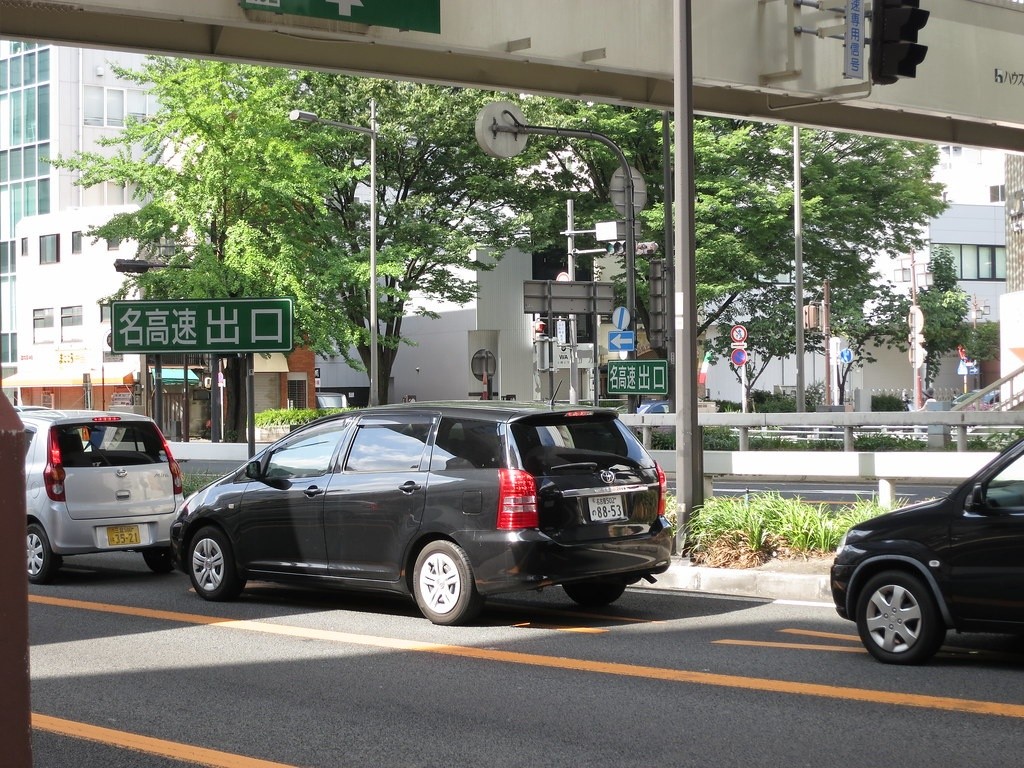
[
  {"left": 952, "top": 388, "right": 999, "bottom": 407},
  {"left": 13, "top": 404, "right": 187, "bottom": 585},
  {"left": 829, "top": 433, "right": 1024, "bottom": 667},
  {"left": 614, "top": 400, "right": 671, "bottom": 415}
]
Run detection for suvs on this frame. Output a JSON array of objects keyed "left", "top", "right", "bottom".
[{"left": 167, "top": 398, "right": 673, "bottom": 627}]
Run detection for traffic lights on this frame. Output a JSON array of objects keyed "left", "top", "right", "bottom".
[
  {"left": 606, "top": 240, "right": 637, "bottom": 254},
  {"left": 870, "top": 1, "right": 929, "bottom": 85}
]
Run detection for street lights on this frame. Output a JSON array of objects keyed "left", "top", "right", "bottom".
[{"left": 289, "top": 96, "right": 381, "bottom": 408}]
